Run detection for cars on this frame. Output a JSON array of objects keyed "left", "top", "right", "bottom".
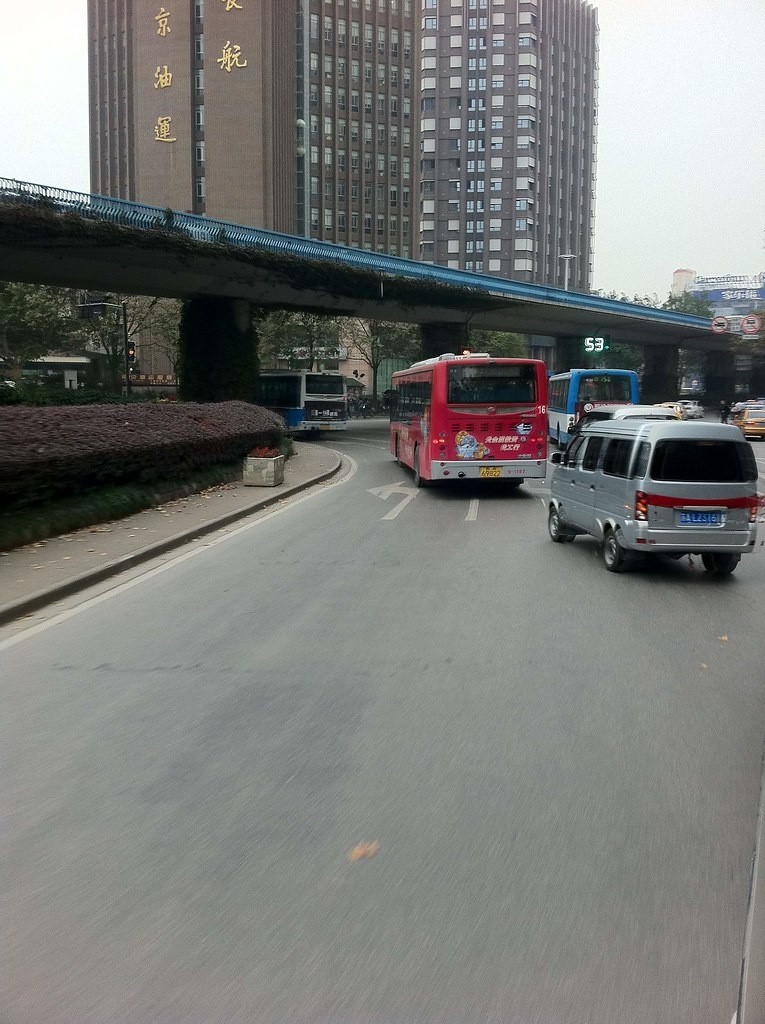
[
  {"left": 730, "top": 410, "right": 765, "bottom": 440},
  {"left": 653, "top": 402, "right": 688, "bottom": 421},
  {"left": 677, "top": 400, "right": 704, "bottom": 418}
]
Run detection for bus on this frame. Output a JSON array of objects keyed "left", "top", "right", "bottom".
[
  {"left": 0, "top": 356, "right": 91, "bottom": 391},
  {"left": 260, "top": 368, "right": 347, "bottom": 432},
  {"left": 548, "top": 369, "right": 639, "bottom": 451},
  {"left": 383, "top": 353, "right": 549, "bottom": 488}
]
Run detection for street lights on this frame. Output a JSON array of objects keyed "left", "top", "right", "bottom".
[{"left": 561, "top": 254, "right": 577, "bottom": 291}]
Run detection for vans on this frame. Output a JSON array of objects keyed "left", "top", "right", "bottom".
[
  {"left": 568, "top": 406, "right": 680, "bottom": 450},
  {"left": 548, "top": 419, "right": 758, "bottom": 576}
]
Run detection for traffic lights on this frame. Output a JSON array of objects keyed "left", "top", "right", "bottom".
[
  {"left": 583, "top": 336, "right": 610, "bottom": 355},
  {"left": 127, "top": 341, "right": 135, "bottom": 362}
]
